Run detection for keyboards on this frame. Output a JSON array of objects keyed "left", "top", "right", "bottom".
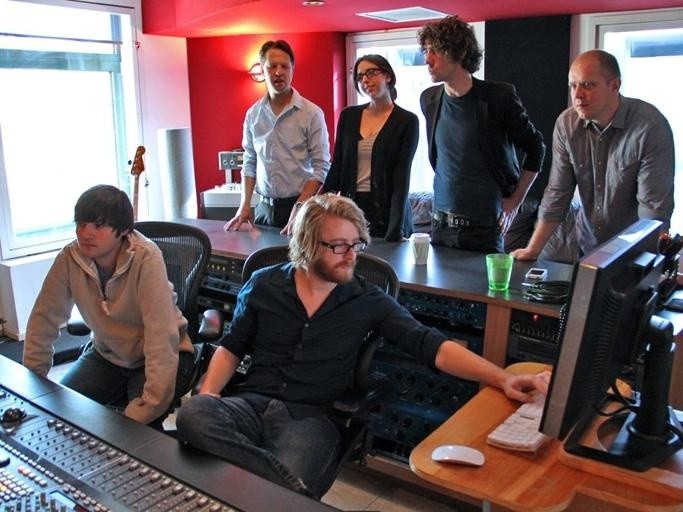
[{"left": 486, "top": 391, "right": 549, "bottom": 453}]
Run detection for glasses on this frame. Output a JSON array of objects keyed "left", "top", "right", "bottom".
[
  {"left": 318, "top": 237, "right": 368, "bottom": 256},
  {"left": 354, "top": 69, "right": 385, "bottom": 84}
]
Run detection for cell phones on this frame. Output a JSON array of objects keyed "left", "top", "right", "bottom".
[
  {"left": 526, "top": 268, "right": 548, "bottom": 280},
  {"left": 667, "top": 299, "right": 683, "bottom": 313}
]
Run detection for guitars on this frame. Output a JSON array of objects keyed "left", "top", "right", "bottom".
[{"left": 131, "top": 146, "right": 145, "bottom": 221}]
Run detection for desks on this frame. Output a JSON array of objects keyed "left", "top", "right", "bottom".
[{"left": 409, "top": 361, "right": 683, "bottom": 512}]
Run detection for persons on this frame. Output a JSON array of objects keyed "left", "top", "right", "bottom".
[
  {"left": 508, "top": 48, "right": 676, "bottom": 264},
  {"left": 20, "top": 183, "right": 196, "bottom": 430},
  {"left": 313, "top": 54, "right": 420, "bottom": 241},
  {"left": 419, "top": 14, "right": 548, "bottom": 252},
  {"left": 223, "top": 39, "right": 331, "bottom": 236},
  {"left": 174, "top": 192, "right": 554, "bottom": 503}
]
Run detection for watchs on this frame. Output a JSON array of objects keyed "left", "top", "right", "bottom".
[{"left": 293, "top": 200, "right": 304, "bottom": 210}]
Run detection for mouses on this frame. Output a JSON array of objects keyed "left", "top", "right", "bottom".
[{"left": 431, "top": 444, "right": 486, "bottom": 466}]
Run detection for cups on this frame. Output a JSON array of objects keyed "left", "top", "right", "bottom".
[
  {"left": 408, "top": 233, "right": 431, "bottom": 264},
  {"left": 485, "top": 253, "right": 513, "bottom": 291}
]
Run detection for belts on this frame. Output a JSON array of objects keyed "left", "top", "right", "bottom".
[
  {"left": 433, "top": 212, "right": 502, "bottom": 229},
  {"left": 263, "top": 197, "right": 299, "bottom": 208}
]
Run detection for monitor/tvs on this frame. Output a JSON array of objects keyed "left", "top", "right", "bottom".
[{"left": 536, "top": 217, "right": 683, "bottom": 474}]
